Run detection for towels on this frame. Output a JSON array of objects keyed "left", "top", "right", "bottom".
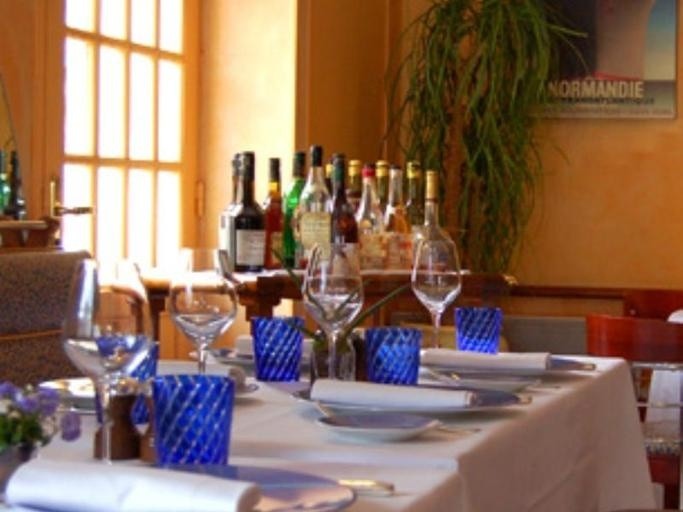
[
  {"left": 233, "top": 337, "right": 319, "bottom": 360},
  {"left": 7, "top": 457, "right": 260, "bottom": 512},
  {"left": 155, "top": 360, "right": 245, "bottom": 392},
  {"left": 310, "top": 375, "right": 479, "bottom": 406},
  {"left": 419, "top": 347, "right": 550, "bottom": 373}
]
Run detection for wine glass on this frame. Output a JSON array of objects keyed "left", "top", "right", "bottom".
[{"left": 61, "top": 237, "right": 463, "bottom": 467}]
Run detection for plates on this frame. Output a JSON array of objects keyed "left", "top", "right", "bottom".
[{"left": 38, "top": 374, "right": 531, "bottom": 512}]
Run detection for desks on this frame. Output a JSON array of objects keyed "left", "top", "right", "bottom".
[{"left": 129, "top": 268, "right": 493, "bottom": 360}]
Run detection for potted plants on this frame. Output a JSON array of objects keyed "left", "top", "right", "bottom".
[{"left": 269, "top": 243, "right": 412, "bottom": 384}]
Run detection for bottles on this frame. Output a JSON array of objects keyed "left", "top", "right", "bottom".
[
  {"left": 0, "top": 150, "right": 11, "bottom": 218},
  {"left": 218, "top": 144, "right": 457, "bottom": 272},
  {"left": 4, "top": 150, "right": 27, "bottom": 220}
]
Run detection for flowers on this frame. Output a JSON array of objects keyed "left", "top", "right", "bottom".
[{"left": 0, "top": 377, "right": 81, "bottom": 462}]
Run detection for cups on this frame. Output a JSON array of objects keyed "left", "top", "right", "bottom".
[{"left": 91, "top": 306, "right": 502, "bottom": 469}]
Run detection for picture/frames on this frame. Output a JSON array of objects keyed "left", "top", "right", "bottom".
[{"left": 475, "top": 0, "right": 676, "bottom": 119}]
